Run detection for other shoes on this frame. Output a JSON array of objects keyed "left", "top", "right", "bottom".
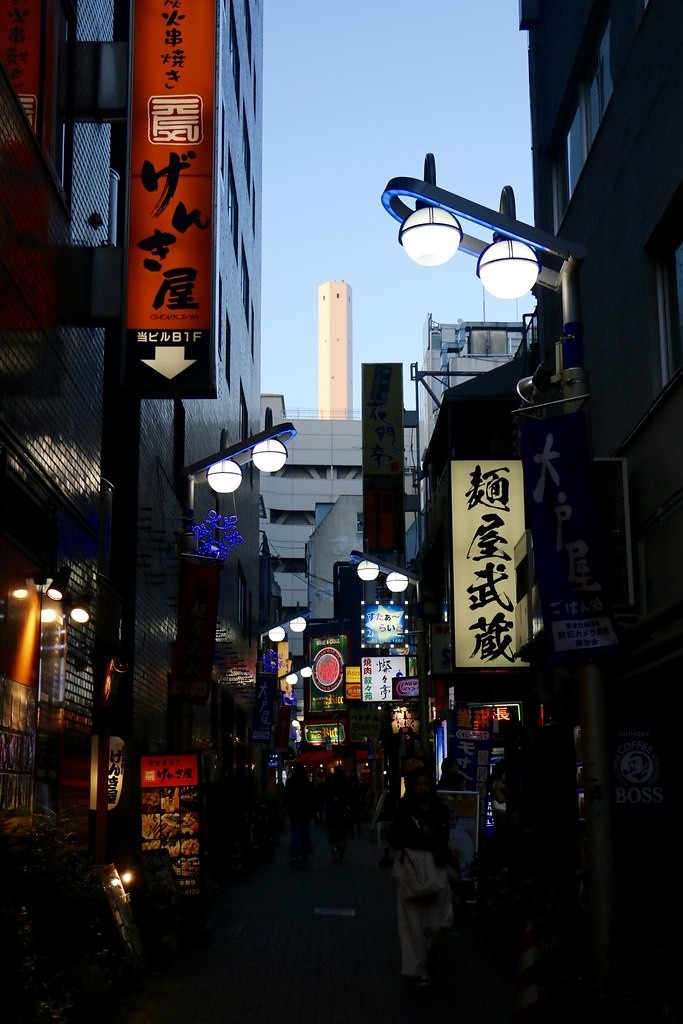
[
  {"left": 292, "top": 857, "right": 298, "bottom": 864},
  {"left": 401, "top": 975, "right": 431, "bottom": 987},
  {"left": 340, "top": 855, "right": 344, "bottom": 863},
  {"left": 333, "top": 851, "right": 338, "bottom": 863}
]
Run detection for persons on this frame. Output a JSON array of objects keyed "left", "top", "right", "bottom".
[{"left": 283, "top": 758, "right": 465, "bottom": 984}]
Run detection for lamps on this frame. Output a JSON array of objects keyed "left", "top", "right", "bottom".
[
  {"left": 66, "top": 591, "right": 92, "bottom": 623},
  {"left": 36, "top": 567, "right": 71, "bottom": 601},
  {"left": 82, "top": 646, "right": 128, "bottom": 673}
]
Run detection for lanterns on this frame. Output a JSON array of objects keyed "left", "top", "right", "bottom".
[{"left": 89, "top": 734, "right": 126, "bottom": 811}]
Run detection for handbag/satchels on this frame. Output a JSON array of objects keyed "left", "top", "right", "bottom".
[{"left": 396, "top": 849, "right": 444, "bottom": 903}]
